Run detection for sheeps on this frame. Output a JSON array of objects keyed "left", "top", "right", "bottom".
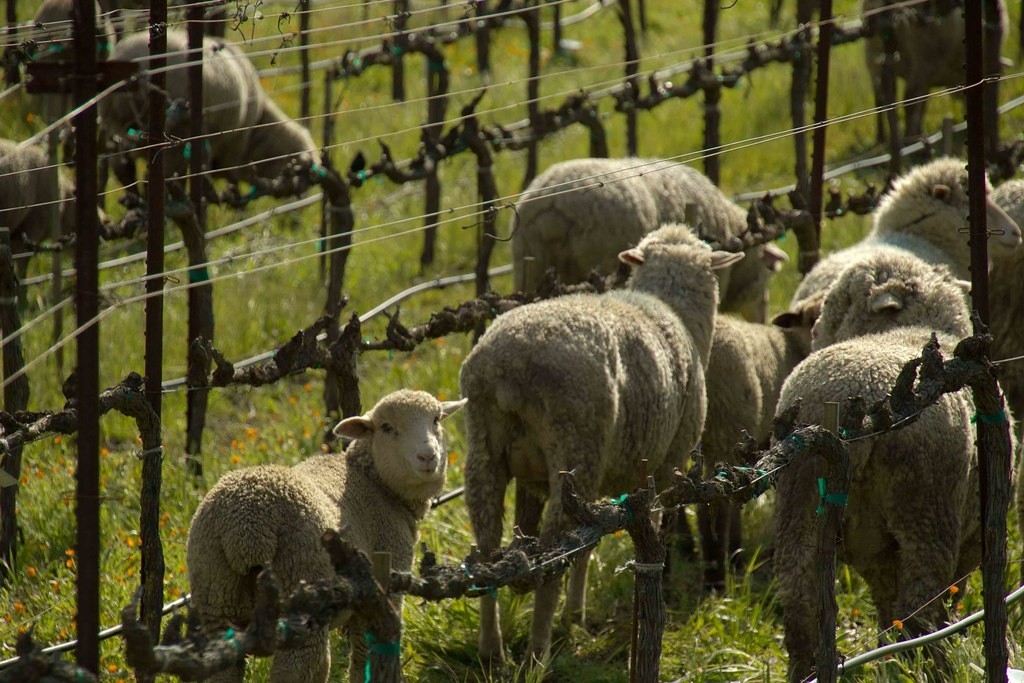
[
  {"left": 0, "top": 0, "right": 323, "bottom": 312},
  {"left": 187, "top": 387, "right": 468, "bottom": 683},
  {"left": 861, "top": 0, "right": 1008, "bottom": 185},
  {"left": 459, "top": 158, "right": 1023, "bottom": 682}
]
